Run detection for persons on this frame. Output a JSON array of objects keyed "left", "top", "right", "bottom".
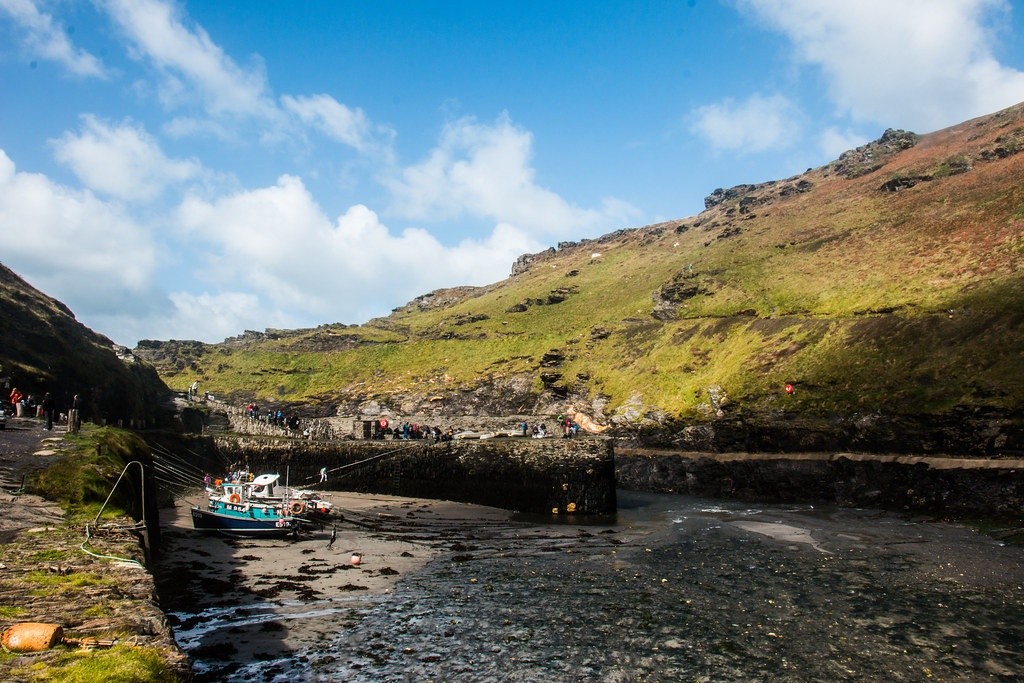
[
  {"left": 279, "top": 507, "right": 292, "bottom": 527},
  {"left": 10, "top": 388, "right": 52, "bottom": 431},
  {"left": 267, "top": 408, "right": 300, "bottom": 429},
  {"left": 249, "top": 504, "right": 254, "bottom": 518},
  {"left": 557, "top": 415, "right": 579, "bottom": 440},
  {"left": 191, "top": 380, "right": 200, "bottom": 395},
  {"left": 263, "top": 506, "right": 271, "bottom": 516},
  {"left": 516, "top": 420, "right": 547, "bottom": 438},
  {"left": 248, "top": 401, "right": 266, "bottom": 423},
  {"left": 394, "top": 422, "right": 454, "bottom": 442},
  {"left": 320, "top": 466, "right": 327, "bottom": 483},
  {"left": 204, "top": 391, "right": 214, "bottom": 401},
  {"left": 73, "top": 395, "right": 80, "bottom": 422},
  {"left": 204, "top": 462, "right": 253, "bottom": 492}
]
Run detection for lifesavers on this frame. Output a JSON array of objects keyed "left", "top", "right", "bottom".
[
  {"left": 230, "top": 493, "right": 240, "bottom": 503},
  {"left": 290, "top": 502, "right": 303, "bottom": 515}
]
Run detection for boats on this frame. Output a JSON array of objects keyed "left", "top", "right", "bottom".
[{"left": 191, "top": 473, "right": 342, "bottom": 538}]
